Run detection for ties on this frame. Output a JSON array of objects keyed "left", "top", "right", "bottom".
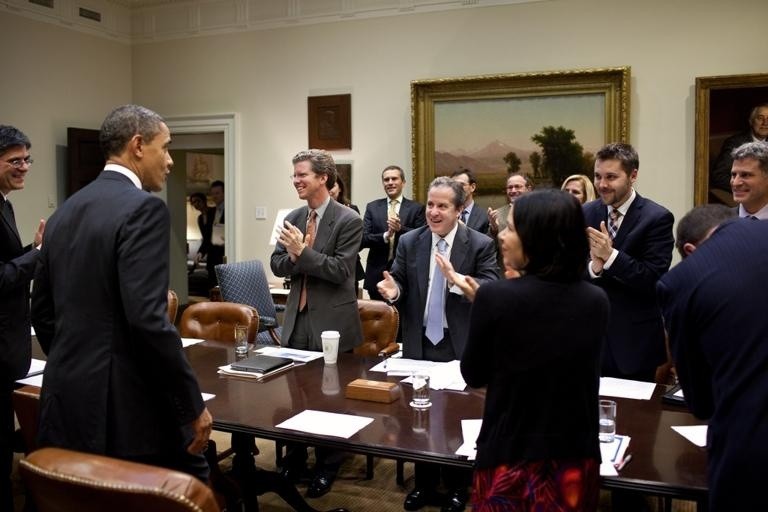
[
  {"left": 299, "top": 210, "right": 317, "bottom": 313},
  {"left": 607, "top": 209, "right": 618, "bottom": 243},
  {"left": 388, "top": 200, "right": 398, "bottom": 262},
  {"left": 425, "top": 239, "right": 446, "bottom": 346},
  {"left": 460, "top": 210, "right": 467, "bottom": 224}
]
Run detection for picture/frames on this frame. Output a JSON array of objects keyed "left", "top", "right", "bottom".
[
  {"left": 409, "top": 65, "right": 631, "bottom": 212},
  {"left": 307, "top": 93, "right": 352, "bottom": 152},
  {"left": 693, "top": 73, "right": 768, "bottom": 209},
  {"left": 334, "top": 163, "right": 351, "bottom": 208}
]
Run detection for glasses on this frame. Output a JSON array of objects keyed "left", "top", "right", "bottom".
[{"left": 13, "top": 160, "right": 32, "bottom": 169}]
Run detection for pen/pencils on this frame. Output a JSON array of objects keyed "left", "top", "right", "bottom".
[{"left": 617, "top": 454, "right": 632, "bottom": 471}]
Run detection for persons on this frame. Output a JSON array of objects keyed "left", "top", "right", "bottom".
[
  {"left": 559, "top": 173, "right": 599, "bottom": 209},
  {"left": 729, "top": 142, "right": 768, "bottom": 226},
  {"left": 655, "top": 205, "right": 768, "bottom": 511},
  {"left": 377, "top": 176, "right": 501, "bottom": 511},
  {"left": 461, "top": 193, "right": 610, "bottom": 512},
  {"left": 189, "top": 192, "right": 215, "bottom": 237},
  {"left": 364, "top": 159, "right": 427, "bottom": 304},
  {"left": 194, "top": 180, "right": 224, "bottom": 296},
  {"left": 446, "top": 163, "right": 492, "bottom": 243},
  {"left": 486, "top": 171, "right": 536, "bottom": 236},
  {"left": 328, "top": 168, "right": 365, "bottom": 309},
  {"left": 0, "top": 123, "right": 46, "bottom": 511},
  {"left": 271, "top": 150, "right": 364, "bottom": 499},
  {"left": 576, "top": 143, "right": 674, "bottom": 379},
  {"left": 29, "top": 102, "right": 214, "bottom": 487},
  {"left": 709, "top": 105, "right": 768, "bottom": 194}
]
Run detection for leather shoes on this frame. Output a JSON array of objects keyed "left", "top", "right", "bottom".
[
  {"left": 275, "top": 463, "right": 337, "bottom": 496},
  {"left": 440, "top": 486, "right": 468, "bottom": 510},
  {"left": 404, "top": 488, "right": 435, "bottom": 510}
]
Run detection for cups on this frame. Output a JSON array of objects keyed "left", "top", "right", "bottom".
[
  {"left": 411, "top": 375, "right": 429, "bottom": 404},
  {"left": 411, "top": 407, "right": 429, "bottom": 434},
  {"left": 597, "top": 400, "right": 616, "bottom": 443},
  {"left": 320, "top": 331, "right": 340, "bottom": 364},
  {"left": 320, "top": 366, "right": 341, "bottom": 396},
  {"left": 233, "top": 326, "right": 247, "bottom": 356}
]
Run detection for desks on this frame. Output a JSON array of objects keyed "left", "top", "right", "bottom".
[{"left": 30, "top": 335, "right": 714, "bottom": 512}]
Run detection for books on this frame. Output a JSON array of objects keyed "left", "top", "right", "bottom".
[{"left": 230, "top": 355, "right": 293, "bottom": 374}]
